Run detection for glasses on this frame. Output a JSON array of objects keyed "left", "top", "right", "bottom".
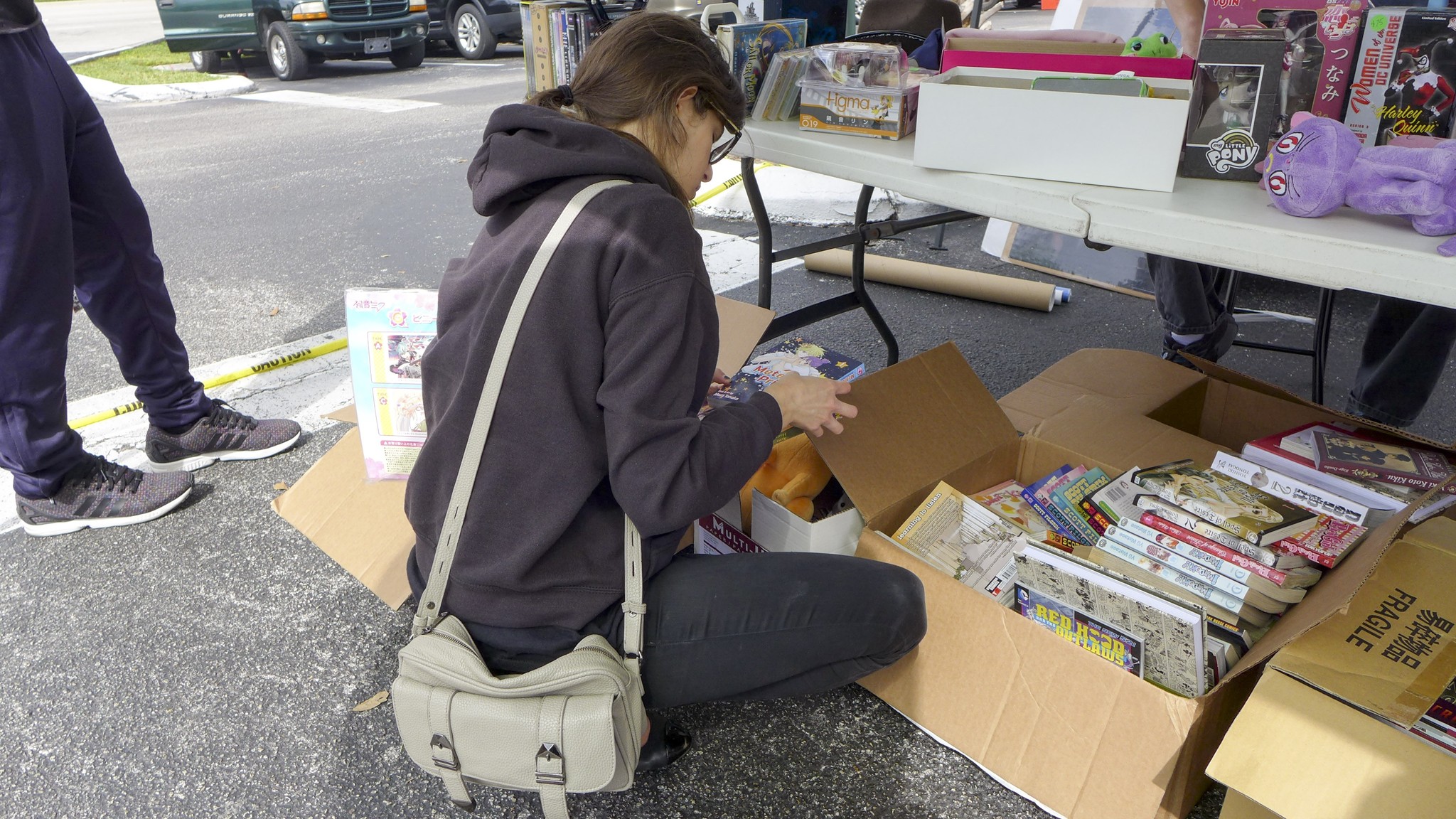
[{"left": 695, "top": 87, "right": 744, "bottom": 165}]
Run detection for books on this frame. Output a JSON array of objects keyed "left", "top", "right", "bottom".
[
  {"left": 890, "top": 413, "right": 1456, "bottom": 752},
  {"left": 695, "top": 337, "right": 866, "bottom": 444},
  {"left": 520, "top": 0, "right": 818, "bottom": 122}
]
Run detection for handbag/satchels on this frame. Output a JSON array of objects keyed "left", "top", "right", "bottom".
[{"left": 389, "top": 617, "right": 645, "bottom": 818}]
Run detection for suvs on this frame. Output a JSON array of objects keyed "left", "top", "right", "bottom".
[
  {"left": 426, "top": 0, "right": 524, "bottom": 61},
  {"left": 156, "top": 0, "right": 431, "bottom": 83}
]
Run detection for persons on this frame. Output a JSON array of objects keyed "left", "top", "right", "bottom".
[
  {"left": 1143, "top": 0, "right": 1456, "bottom": 428},
  {"left": 406, "top": 11, "right": 926, "bottom": 775},
  {"left": 0, "top": 0, "right": 303, "bottom": 535}
]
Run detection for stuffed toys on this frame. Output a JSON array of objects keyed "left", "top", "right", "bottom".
[
  {"left": 1254, "top": 111, "right": 1456, "bottom": 258},
  {"left": 1120, "top": 32, "right": 1177, "bottom": 58},
  {"left": 739, "top": 434, "right": 832, "bottom": 539}
]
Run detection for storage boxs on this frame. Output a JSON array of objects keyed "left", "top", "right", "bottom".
[
  {"left": 798, "top": 0, "right": 1456, "bottom": 194},
  {"left": 268, "top": 293, "right": 1455, "bottom": 818}
]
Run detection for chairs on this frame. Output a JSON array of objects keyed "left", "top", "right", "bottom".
[
  {"left": 1207, "top": 260, "right": 1334, "bottom": 407},
  {"left": 857, "top": 0, "right": 963, "bottom": 38}
]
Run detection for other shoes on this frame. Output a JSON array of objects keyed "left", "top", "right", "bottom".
[
  {"left": 630, "top": 715, "right": 693, "bottom": 770},
  {"left": 1163, "top": 314, "right": 1238, "bottom": 372}
]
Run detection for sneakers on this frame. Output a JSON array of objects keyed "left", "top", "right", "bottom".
[
  {"left": 17, "top": 453, "right": 195, "bottom": 536},
  {"left": 144, "top": 398, "right": 300, "bottom": 472}
]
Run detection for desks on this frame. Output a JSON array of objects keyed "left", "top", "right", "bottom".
[{"left": 711, "top": 118, "right": 1456, "bottom": 368}]
